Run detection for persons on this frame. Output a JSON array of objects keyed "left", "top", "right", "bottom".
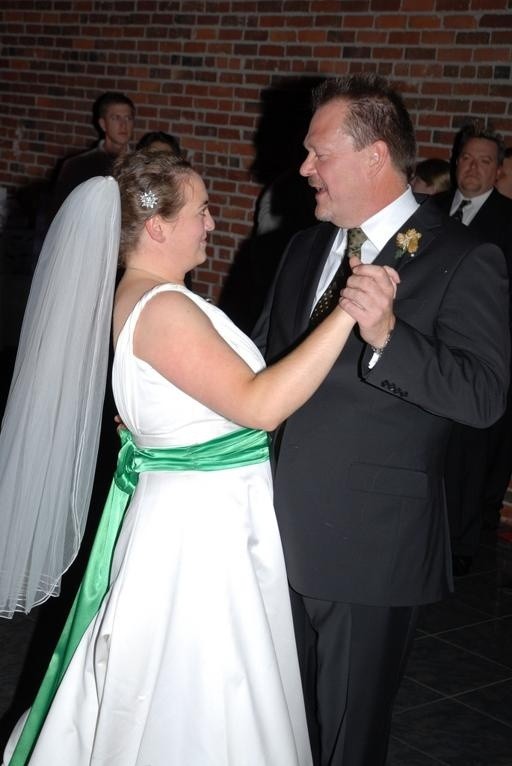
[
  {"left": 409, "top": 129, "right": 512, "bottom": 226},
  {"left": 57, "top": 92, "right": 181, "bottom": 180},
  {"left": 115, "top": 75, "right": 512, "bottom": 766},
  {"left": 0, "top": 151, "right": 401, "bottom": 766}
]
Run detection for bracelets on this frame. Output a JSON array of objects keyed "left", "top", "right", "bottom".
[{"left": 371, "top": 327, "right": 394, "bottom": 356}]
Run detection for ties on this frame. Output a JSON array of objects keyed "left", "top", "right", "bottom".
[
  {"left": 448, "top": 199, "right": 471, "bottom": 225},
  {"left": 308, "top": 228, "right": 368, "bottom": 337}
]
